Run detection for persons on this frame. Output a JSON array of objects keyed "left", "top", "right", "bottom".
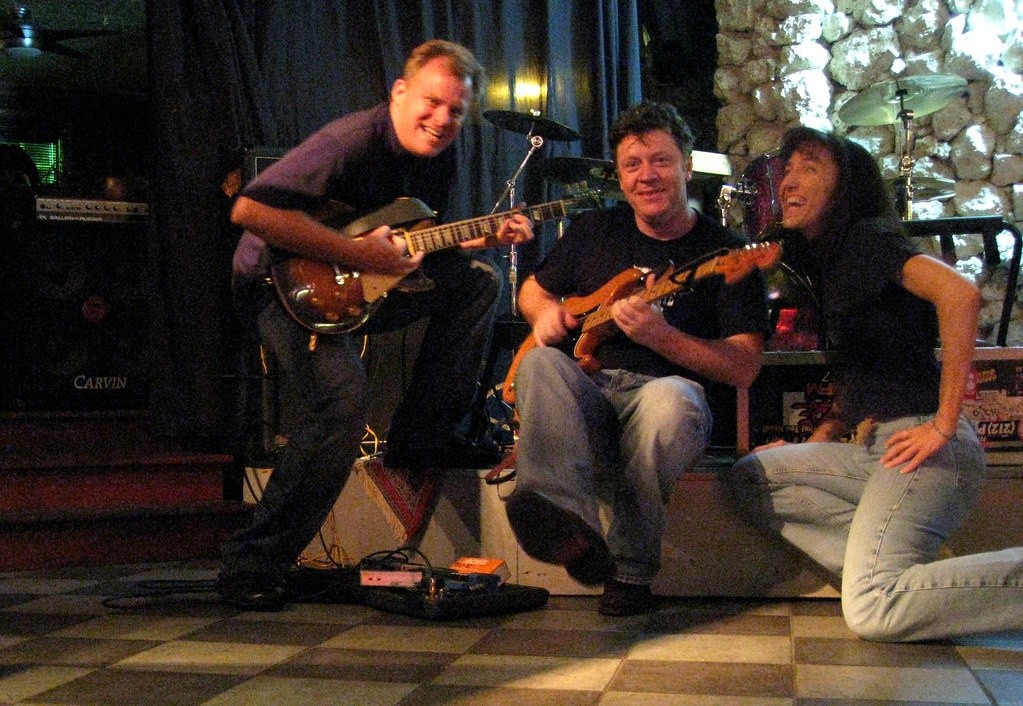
[
  {"left": 216, "top": 40, "right": 534, "bottom": 613},
  {"left": 504, "top": 100, "right": 772, "bottom": 614},
  {"left": 727, "top": 125, "right": 1023, "bottom": 643}
]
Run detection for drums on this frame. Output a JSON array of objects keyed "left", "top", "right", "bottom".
[
  {"left": 760, "top": 258, "right": 822, "bottom": 339},
  {"left": 739, "top": 147, "right": 788, "bottom": 244}
]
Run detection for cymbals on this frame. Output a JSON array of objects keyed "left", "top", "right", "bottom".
[
  {"left": 534, "top": 154, "right": 630, "bottom": 203},
  {"left": 838, "top": 72, "right": 969, "bottom": 127},
  {"left": 481, "top": 108, "right": 581, "bottom": 142}
]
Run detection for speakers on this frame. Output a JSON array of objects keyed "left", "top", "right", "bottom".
[{"left": 238, "top": 145, "right": 430, "bottom": 462}]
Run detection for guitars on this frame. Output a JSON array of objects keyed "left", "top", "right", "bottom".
[
  {"left": 502, "top": 239, "right": 784, "bottom": 406},
  {"left": 269, "top": 185, "right": 609, "bottom": 336}
]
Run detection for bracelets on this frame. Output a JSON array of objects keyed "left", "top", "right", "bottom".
[{"left": 932, "top": 418, "right": 956, "bottom": 438}]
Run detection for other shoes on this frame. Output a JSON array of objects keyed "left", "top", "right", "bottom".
[
  {"left": 597, "top": 580, "right": 653, "bottom": 615},
  {"left": 215, "top": 574, "right": 283, "bottom": 611},
  {"left": 506, "top": 491, "right": 614, "bottom": 588},
  {"left": 384, "top": 427, "right": 502, "bottom": 468}
]
